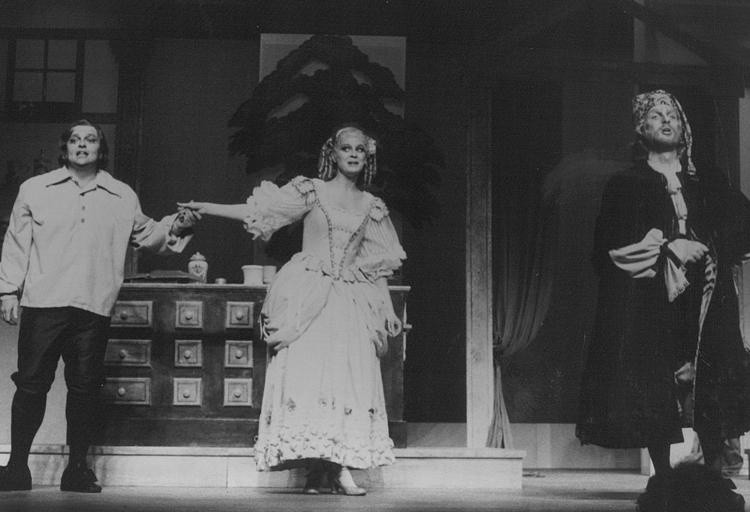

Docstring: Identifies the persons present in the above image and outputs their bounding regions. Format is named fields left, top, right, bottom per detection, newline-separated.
left=0, top=117, right=204, bottom=494
left=634, top=459, right=746, bottom=511
left=571, top=86, right=750, bottom=476
left=175, top=124, right=410, bottom=498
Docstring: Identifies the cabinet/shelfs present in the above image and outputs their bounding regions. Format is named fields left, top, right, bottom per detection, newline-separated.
left=97, top=282, right=408, bottom=445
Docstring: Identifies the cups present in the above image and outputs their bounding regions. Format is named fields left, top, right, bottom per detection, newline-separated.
left=240, top=264, right=277, bottom=286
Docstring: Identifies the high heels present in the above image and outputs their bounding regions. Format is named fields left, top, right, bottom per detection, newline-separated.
left=303, top=470, right=324, bottom=495
left=328, top=467, right=367, bottom=495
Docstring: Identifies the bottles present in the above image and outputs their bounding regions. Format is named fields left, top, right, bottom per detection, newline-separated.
left=187, top=250, right=209, bottom=285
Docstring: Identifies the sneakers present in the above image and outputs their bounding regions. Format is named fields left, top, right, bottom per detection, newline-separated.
left=60, top=466, right=102, bottom=493
left=2, top=464, right=33, bottom=491
